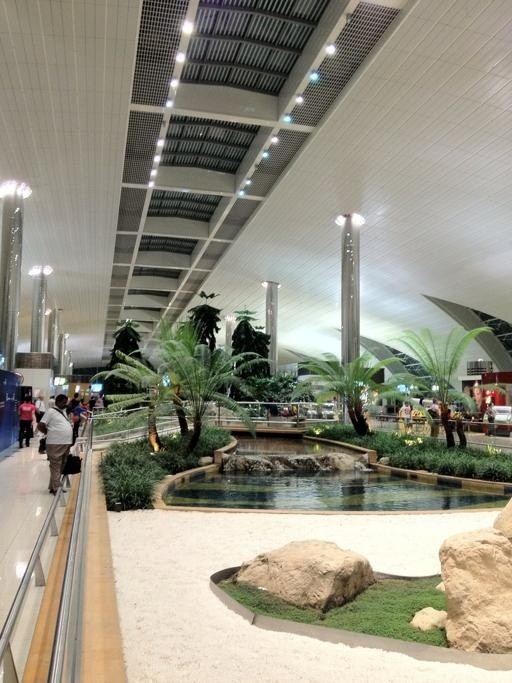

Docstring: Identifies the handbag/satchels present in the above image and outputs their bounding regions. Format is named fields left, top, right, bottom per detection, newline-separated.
left=61, top=454, right=83, bottom=474
left=38, top=436, right=47, bottom=455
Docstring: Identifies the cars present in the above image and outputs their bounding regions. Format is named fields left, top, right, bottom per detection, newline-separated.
left=307, top=404, right=334, bottom=418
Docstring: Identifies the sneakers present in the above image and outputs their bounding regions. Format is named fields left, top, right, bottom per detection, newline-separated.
left=50, top=488, right=68, bottom=495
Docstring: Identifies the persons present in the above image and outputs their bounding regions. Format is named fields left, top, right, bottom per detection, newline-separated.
left=17, top=392, right=104, bottom=447
left=37, top=394, right=73, bottom=493
left=485, top=401, right=497, bottom=435
left=399, top=400, right=414, bottom=434
left=430, top=396, right=441, bottom=436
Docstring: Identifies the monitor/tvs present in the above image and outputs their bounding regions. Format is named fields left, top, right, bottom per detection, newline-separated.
left=91, top=383, right=103, bottom=393
